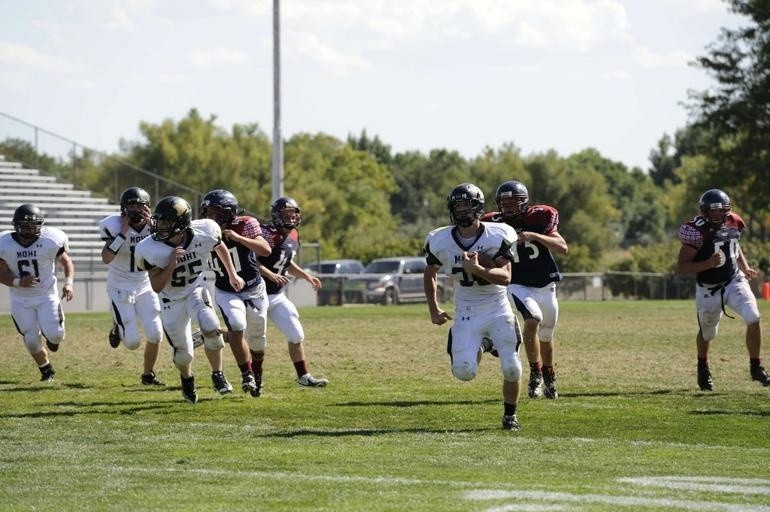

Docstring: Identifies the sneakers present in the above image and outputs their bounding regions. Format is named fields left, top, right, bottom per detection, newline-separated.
left=541, top=367, right=558, bottom=399
left=502, top=416, right=520, bottom=431
left=528, top=372, right=544, bottom=398
left=481, top=332, right=499, bottom=357
left=110, top=319, right=330, bottom=404
left=751, top=367, right=770, bottom=387
left=47, top=339, right=60, bottom=352
left=40, top=370, right=56, bottom=381
left=698, top=373, right=714, bottom=390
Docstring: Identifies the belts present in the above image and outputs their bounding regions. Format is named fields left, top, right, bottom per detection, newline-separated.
left=696, top=268, right=740, bottom=319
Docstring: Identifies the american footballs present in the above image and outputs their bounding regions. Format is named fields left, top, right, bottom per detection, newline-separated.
left=467, top=250, right=498, bottom=269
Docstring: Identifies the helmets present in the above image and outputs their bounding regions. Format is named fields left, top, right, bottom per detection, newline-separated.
left=446, top=183, right=485, bottom=228
left=12, top=204, right=43, bottom=238
left=699, top=189, right=732, bottom=228
left=495, top=181, right=529, bottom=216
left=120, top=187, right=302, bottom=242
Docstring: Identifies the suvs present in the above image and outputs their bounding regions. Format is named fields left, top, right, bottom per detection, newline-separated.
left=305, top=257, right=363, bottom=304
left=364, top=258, right=452, bottom=305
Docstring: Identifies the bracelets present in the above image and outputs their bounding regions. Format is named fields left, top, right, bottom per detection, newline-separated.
left=62, top=276, right=73, bottom=285
left=12, top=278, right=21, bottom=287
left=108, top=234, right=126, bottom=256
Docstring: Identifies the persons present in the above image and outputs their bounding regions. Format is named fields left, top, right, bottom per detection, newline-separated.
left=200, top=190, right=273, bottom=395
left=676, top=189, right=770, bottom=390
left=421, top=183, right=523, bottom=433
left=134, top=197, right=246, bottom=405
left=0, top=205, right=74, bottom=386
left=190, top=199, right=330, bottom=389
left=99, top=187, right=168, bottom=386
left=479, top=181, right=568, bottom=399
